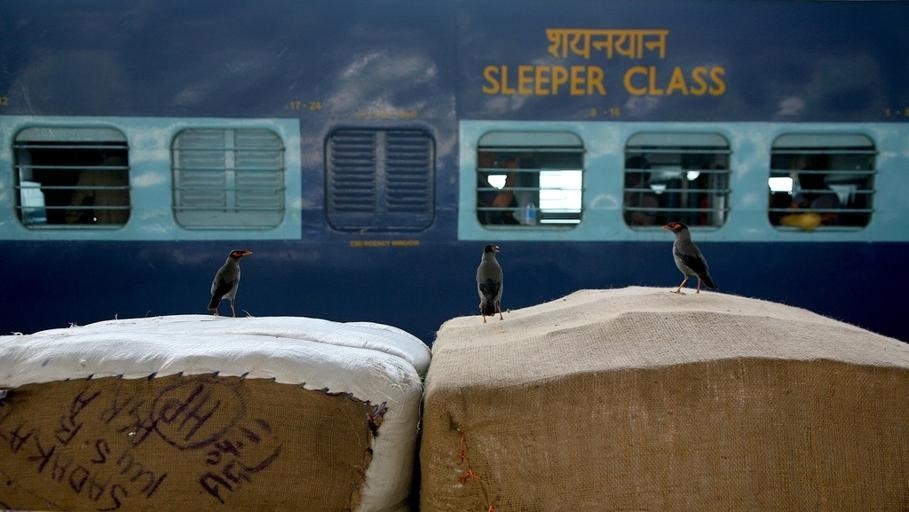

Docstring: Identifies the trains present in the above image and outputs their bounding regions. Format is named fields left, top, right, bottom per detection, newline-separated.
left=0, top=2, right=908, bottom=344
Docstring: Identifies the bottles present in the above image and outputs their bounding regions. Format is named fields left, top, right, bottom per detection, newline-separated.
left=520, top=200, right=536, bottom=225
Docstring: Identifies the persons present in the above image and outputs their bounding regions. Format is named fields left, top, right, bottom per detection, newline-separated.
left=476, top=145, right=520, bottom=226
left=770, top=164, right=841, bottom=227
left=623, top=156, right=727, bottom=226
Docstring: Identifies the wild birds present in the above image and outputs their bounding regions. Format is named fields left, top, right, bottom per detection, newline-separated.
left=476, top=245, right=505, bottom=324
left=208, top=249, right=253, bottom=317
left=661, top=221, right=716, bottom=296
